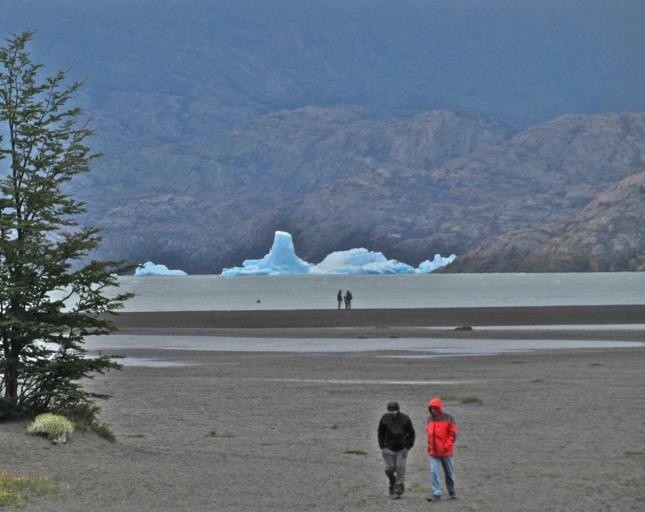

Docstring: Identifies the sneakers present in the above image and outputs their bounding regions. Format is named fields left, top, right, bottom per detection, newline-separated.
left=427, top=495, right=440, bottom=502
left=389, top=483, right=404, bottom=494
left=449, top=489, right=456, bottom=498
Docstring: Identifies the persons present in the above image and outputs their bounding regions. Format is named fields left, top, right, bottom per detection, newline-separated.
left=337, top=290, right=342, bottom=311
left=424, top=397, right=459, bottom=501
left=378, top=401, right=416, bottom=499
left=344, top=291, right=353, bottom=312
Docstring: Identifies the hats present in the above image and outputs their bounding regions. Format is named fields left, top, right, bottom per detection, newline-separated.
left=387, top=403, right=399, bottom=411
